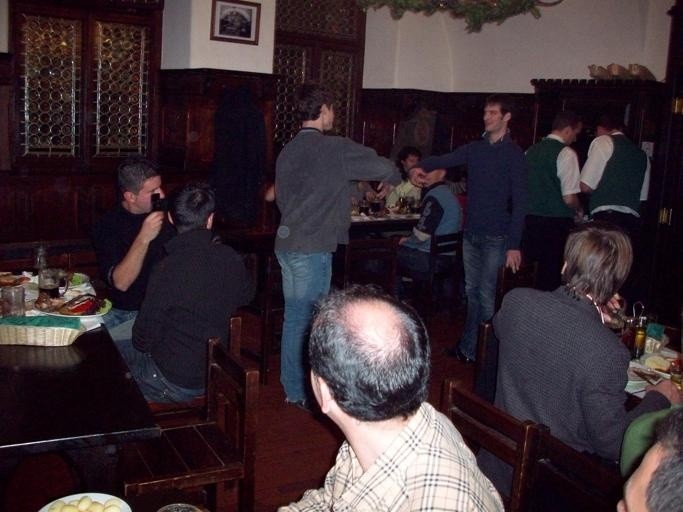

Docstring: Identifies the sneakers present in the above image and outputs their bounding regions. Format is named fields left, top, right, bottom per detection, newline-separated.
left=445, top=345, right=475, bottom=365
left=287, top=398, right=315, bottom=412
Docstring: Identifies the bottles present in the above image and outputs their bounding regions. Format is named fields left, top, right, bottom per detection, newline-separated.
left=622, top=316, right=648, bottom=359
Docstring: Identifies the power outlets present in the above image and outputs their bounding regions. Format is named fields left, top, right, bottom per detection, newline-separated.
left=641, top=140, right=655, bottom=158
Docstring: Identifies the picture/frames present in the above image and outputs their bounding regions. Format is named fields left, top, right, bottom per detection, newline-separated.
left=209, top=0, right=261, bottom=46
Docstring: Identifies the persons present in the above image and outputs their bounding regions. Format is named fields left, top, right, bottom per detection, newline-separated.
left=477, top=218, right=683, bottom=512
left=113, top=179, right=256, bottom=404
left=277, top=282, right=505, bottom=512
left=580, top=113, right=647, bottom=231
left=616, top=407, right=683, bottom=512
left=525, top=110, right=585, bottom=290
left=63, top=157, right=179, bottom=340
left=265, top=145, right=468, bottom=302
left=274, top=84, right=403, bottom=417
left=407, top=92, right=529, bottom=363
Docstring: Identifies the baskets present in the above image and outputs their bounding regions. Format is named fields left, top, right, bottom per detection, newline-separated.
left=1, top=316, right=87, bottom=348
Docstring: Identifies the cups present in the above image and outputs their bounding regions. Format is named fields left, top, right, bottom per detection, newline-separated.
left=0, top=285, right=26, bottom=317
left=670, top=359, right=683, bottom=391
left=601, top=296, right=628, bottom=329
left=30, top=240, right=71, bottom=300
left=157, top=502, right=202, bottom=512
left=357, top=199, right=369, bottom=216
left=371, top=200, right=381, bottom=213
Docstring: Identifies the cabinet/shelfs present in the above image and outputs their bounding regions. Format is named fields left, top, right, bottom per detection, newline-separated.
left=528, top=75, right=665, bottom=210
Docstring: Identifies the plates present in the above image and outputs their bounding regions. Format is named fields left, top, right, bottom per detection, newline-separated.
left=386, top=214, right=421, bottom=218
left=37, top=492, right=132, bottom=512
left=629, top=360, right=671, bottom=380
left=30, top=270, right=109, bottom=317
